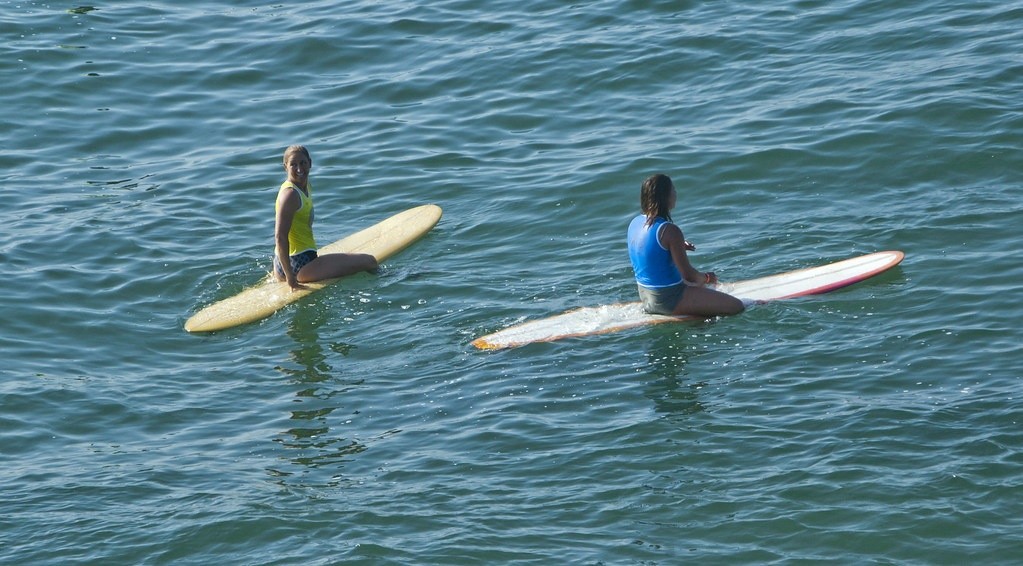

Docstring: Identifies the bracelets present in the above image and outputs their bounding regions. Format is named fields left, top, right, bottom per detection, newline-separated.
left=703, top=271, right=710, bottom=283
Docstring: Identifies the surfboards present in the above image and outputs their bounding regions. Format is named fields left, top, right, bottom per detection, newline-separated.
left=469, top=247, right=906, bottom=355
left=181, top=202, right=444, bottom=336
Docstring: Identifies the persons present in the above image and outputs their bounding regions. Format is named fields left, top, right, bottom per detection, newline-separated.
left=273, top=145, right=383, bottom=292
left=627, top=174, right=746, bottom=316
